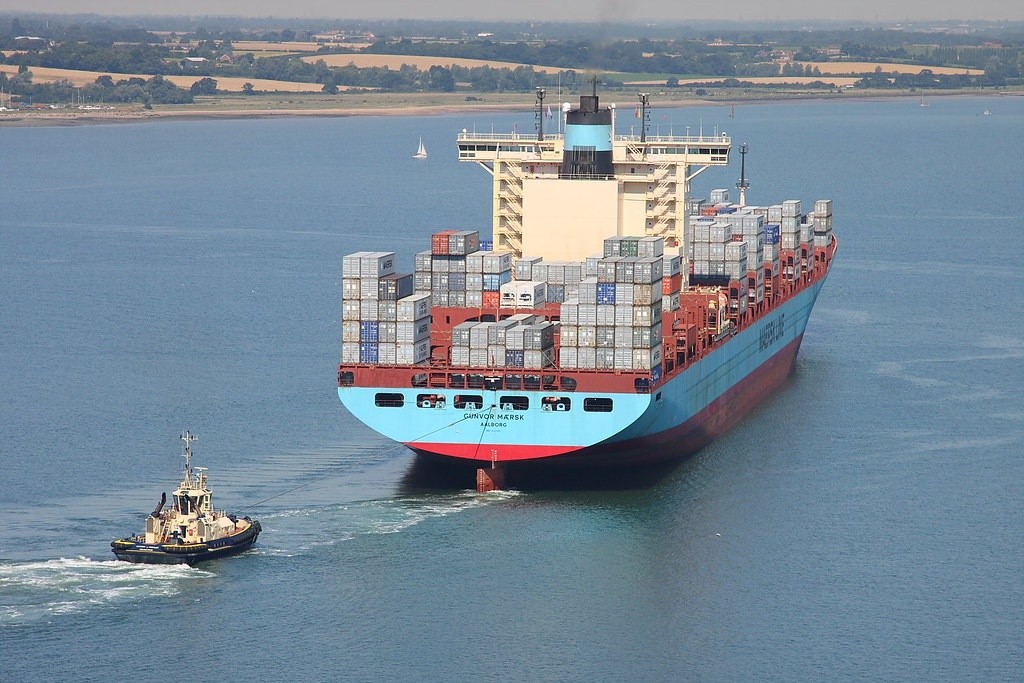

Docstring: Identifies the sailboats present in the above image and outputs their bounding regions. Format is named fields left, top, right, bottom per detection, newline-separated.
left=412, top=136, right=428, bottom=160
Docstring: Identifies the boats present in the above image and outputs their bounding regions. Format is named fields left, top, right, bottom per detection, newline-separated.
left=111, top=431, right=263, bottom=565
left=337, top=70, right=839, bottom=495
